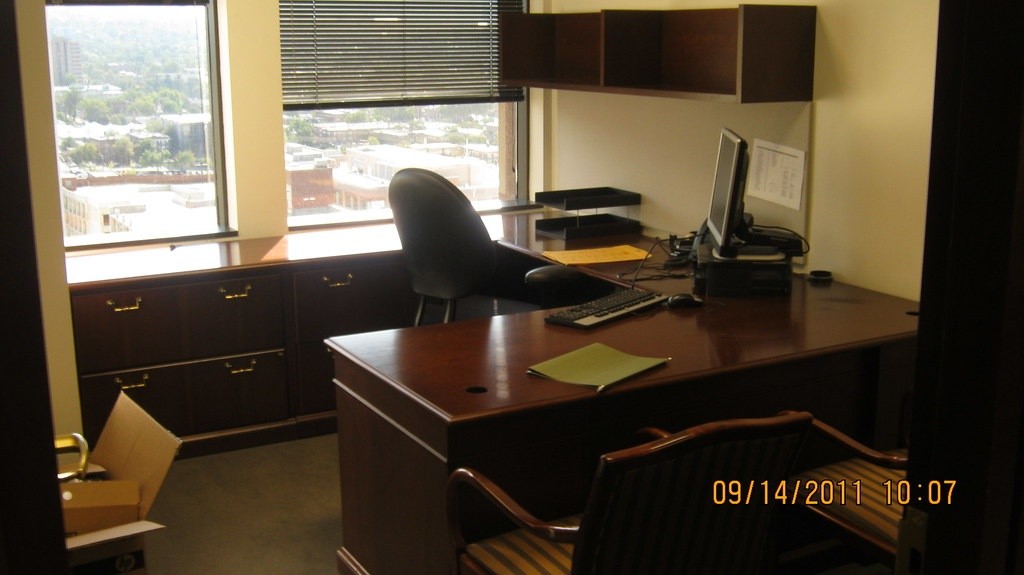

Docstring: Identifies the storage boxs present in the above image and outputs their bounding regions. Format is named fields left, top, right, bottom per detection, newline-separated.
left=54, top=390, right=182, bottom=575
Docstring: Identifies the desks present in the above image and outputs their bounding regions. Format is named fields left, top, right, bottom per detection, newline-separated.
left=325, top=225, right=918, bottom=575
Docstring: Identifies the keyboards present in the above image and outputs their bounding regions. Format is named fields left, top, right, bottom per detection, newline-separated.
left=544, top=288, right=668, bottom=330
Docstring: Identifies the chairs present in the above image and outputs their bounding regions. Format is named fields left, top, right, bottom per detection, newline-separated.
left=449, top=413, right=929, bottom=575
left=385, top=168, right=577, bottom=330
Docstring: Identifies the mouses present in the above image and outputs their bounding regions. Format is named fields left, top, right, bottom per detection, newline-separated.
left=668, top=293, right=704, bottom=309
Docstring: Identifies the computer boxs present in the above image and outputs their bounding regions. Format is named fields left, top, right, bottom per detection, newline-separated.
left=693, top=244, right=793, bottom=300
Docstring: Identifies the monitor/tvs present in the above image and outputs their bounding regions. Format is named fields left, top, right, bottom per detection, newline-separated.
left=706, top=128, right=804, bottom=260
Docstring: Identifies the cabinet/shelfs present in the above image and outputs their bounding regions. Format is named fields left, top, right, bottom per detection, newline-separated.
left=62, top=203, right=589, bottom=468
left=498, top=5, right=816, bottom=103
